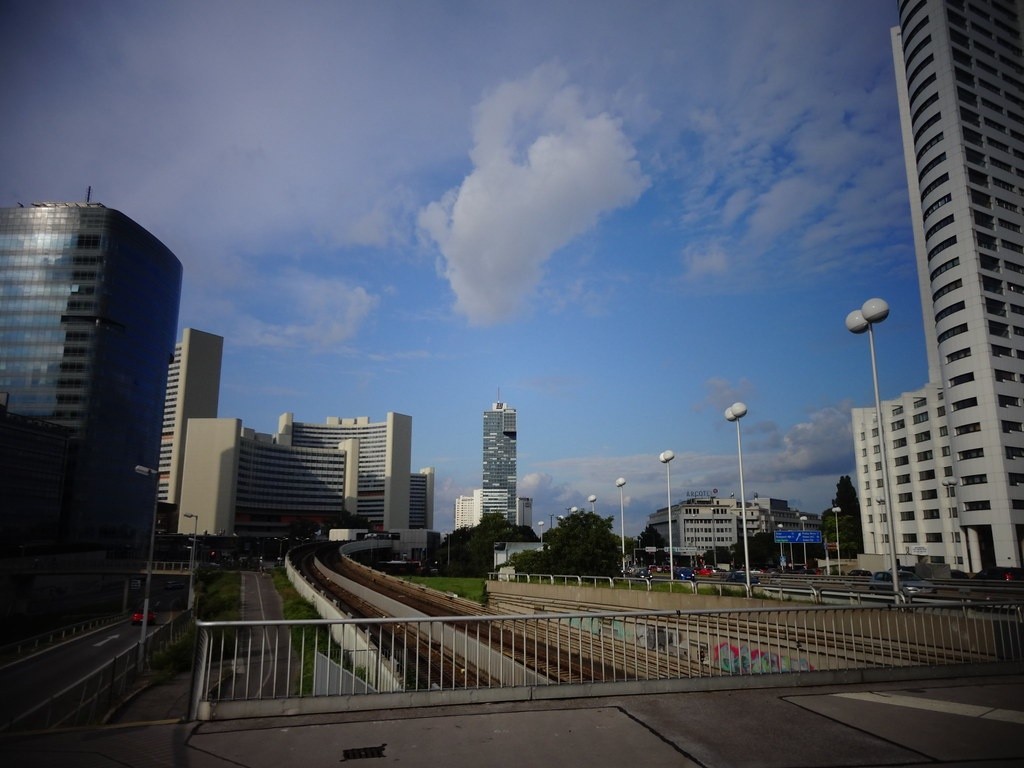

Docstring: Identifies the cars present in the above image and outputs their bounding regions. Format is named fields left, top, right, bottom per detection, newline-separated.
left=964, top=566, right=1024, bottom=609
left=130, top=606, right=156, bottom=625
left=622, top=564, right=970, bottom=607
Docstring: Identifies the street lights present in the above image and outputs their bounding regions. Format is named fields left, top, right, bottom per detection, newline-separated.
left=942, top=477, right=961, bottom=568
left=134, top=464, right=160, bottom=671
left=274, top=537, right=288, bottom=558
left=659, top=449, right=674, bottom=584
left=616, top=476, right=626, bottom=581
left=724, top=402, right=754, bottom=597
left=799, top=515, right=809, bottom=575
left=832, top=506, right=843, bottom=576
left=182, top=512, right=200, bottom=612
left=295, top=537, right=309, bottom=544
left=778, top=523, right=785, bottom=571
left=538, top=520, right=545, bottom=546
left=549, top=513, right=554, bottom=528
left=565, top=506, right=570, bottom=515
left=845, top=297, right=902, bottom=611
left=710, top=507, right=716, bottom=568
left=587, top=495, right=597, bottom=515
